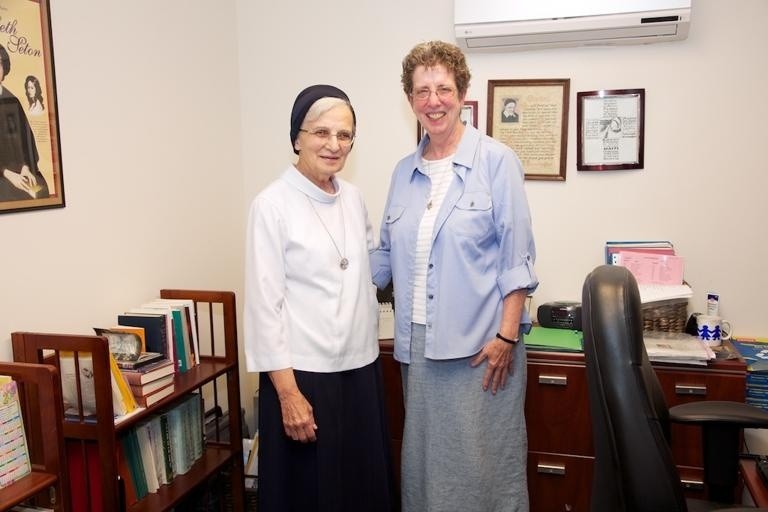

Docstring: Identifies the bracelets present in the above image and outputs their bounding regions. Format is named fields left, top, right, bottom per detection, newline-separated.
left=495, top=331, right=522, bottom=343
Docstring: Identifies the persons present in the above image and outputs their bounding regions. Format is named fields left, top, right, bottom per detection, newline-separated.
left=501, top=97, right=521, bottom=125
left=244, top=84, right=402, bottom=510
left=365, top=41, right=539, bottom=511
left=25, top=74, right=44, bottom=115
left=1, top=48, right=50, bottom=201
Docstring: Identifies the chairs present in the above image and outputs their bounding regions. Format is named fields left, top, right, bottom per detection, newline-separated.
left=582, top=265, right=768, bottom=512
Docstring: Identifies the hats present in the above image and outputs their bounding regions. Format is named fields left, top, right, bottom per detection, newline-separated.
left=290, top=85, right=356, bottom=155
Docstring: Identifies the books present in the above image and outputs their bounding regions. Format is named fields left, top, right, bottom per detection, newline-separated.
left=730, top=336, right=768, bottom=409
left=59, top=298, right=207, bottom=511
left=605, top=240, right=684, bottom=286
left=0, top=375, right=32, bottom=492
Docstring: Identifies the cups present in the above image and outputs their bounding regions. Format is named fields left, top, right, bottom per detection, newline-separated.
left=696, top=315, right=733, bottom=347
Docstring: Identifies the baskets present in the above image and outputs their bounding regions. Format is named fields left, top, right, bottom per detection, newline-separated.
left=222, top=487, right=258, bottom=512
left=642, top=281, right=690, bottom=333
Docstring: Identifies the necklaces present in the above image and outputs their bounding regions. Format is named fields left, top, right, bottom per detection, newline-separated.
left=306, top=197, right=352, bottom=271
left=428, top=144, right=453, bottom=208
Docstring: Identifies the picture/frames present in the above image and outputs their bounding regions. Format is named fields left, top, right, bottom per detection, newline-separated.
left=0, top=0, right=66, bottom=215
left=486, top=78, right=570, bottom=181
left=417, top=101, right=478, bottom=147
left=577, top=88, right=645, bottom=171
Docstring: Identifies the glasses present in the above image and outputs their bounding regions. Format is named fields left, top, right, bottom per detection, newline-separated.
left=298, top=128, right=355, bottom=145
left=410, top=85, right=456, bottom=99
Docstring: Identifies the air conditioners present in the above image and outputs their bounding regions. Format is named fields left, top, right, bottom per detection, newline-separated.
left=453, top=0, right=692, bottom=55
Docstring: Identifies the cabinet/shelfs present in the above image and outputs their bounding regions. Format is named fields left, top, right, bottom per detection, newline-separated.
left=11, top=288, right=246, bottom=512
left=378, top=337, right=748, bottom=512
left=0, top=361, right=72, bottom=512
